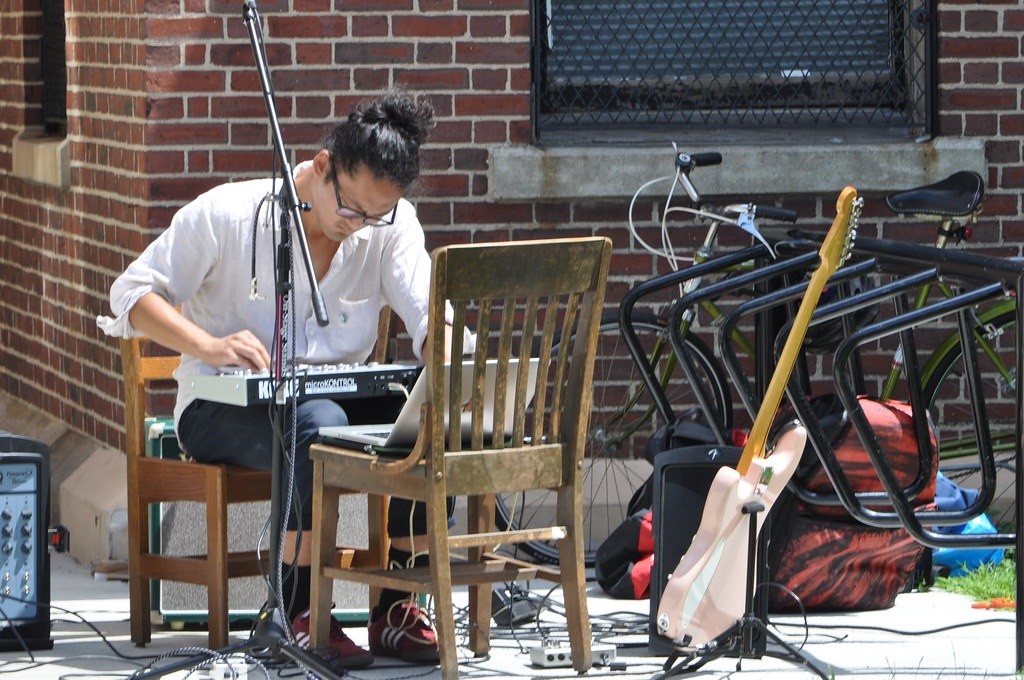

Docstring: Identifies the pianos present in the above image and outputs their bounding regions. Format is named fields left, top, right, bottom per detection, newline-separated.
left=188, top=359, right=428, bottom=408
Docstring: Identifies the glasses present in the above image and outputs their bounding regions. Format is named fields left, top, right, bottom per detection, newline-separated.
left=326, top=154, right=399, bottom=228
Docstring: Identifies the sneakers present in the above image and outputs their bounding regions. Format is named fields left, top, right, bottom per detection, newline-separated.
left=291, top=603, right=374, bottom=667
left=368, top=604, right=441, bottom=661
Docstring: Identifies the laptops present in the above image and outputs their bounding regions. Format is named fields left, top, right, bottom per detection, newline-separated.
left=318, top=358, right=554, bottom=447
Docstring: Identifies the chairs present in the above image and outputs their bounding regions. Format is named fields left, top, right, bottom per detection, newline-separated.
left=308, top=236, right=616, bottom=676
left=117, top=299, right=400, bottom=650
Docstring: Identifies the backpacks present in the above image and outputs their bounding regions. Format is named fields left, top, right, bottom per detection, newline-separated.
left=766, top=392, right=937, bottom=615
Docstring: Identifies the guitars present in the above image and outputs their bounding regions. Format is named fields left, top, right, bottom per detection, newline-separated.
left=654, top=183, right=865, bottom=654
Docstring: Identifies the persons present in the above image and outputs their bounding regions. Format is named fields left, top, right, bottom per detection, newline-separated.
left=96, top=87, right=480, bottom=669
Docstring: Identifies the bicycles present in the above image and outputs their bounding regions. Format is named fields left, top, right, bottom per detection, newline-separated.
left=482, top=148, right=1024, bottom=572
